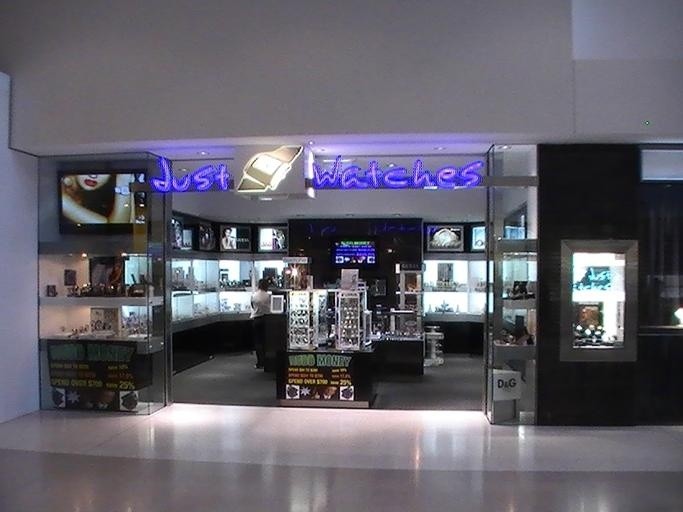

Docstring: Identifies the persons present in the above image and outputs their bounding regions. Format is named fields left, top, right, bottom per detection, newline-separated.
left=250, top=279, right=271, bottom=368
left=60, top=173, right=135, bottom=223
left=222, top=229, right=237, bottom=249
left=312, top=386, right=337, bottom=400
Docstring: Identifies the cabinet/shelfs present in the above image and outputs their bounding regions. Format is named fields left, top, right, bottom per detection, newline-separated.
left=38, top=176, right=537, bottom=360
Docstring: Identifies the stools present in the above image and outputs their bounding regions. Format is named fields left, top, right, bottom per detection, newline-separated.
left=424, top=325, right=444, bottom=368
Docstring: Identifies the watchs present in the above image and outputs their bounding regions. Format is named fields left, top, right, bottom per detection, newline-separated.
left=113, top=186, right=131, bottom=195
left=575, top=324, right=616, bottom=345
